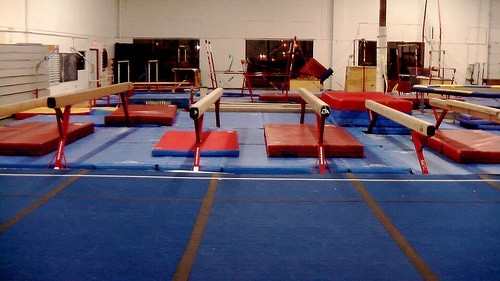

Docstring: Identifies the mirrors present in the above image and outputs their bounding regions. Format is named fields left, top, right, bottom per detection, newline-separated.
left=59, top=52, right=78, bottom=85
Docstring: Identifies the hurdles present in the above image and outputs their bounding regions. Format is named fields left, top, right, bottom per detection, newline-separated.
left=296, top=86, right=335, bottom=173
left=362, top=96, right=437, bottom=177
left=427, top=95, right=499, bottom=128
left=43, top=81, right=134, bottom=169
left=188, top=86, right=226, bottom=169
